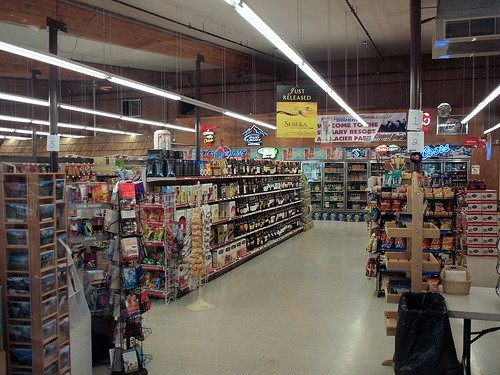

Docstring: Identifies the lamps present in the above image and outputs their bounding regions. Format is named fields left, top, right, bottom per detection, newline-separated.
left=460, top=18, right=500, bottom=134
left=223, top=0, right=369, bottom=128
left=0, top=0, right=276, bottom=141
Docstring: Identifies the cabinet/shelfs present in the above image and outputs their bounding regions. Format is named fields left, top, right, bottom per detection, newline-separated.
left=374, top=186, right=459, bottom=297
left=381, top=172, right=439, bottom=366
left=0, top=170, right=153, bottom=375
left=154, top=182, right=247, bottom=285
left=141, top=173, right=304, bottom=298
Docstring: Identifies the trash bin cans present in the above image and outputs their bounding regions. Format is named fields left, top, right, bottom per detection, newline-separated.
left=392, top=292, right=462, bottom=375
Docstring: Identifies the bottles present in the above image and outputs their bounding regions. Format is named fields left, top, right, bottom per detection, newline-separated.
left=235, top=190, right=300, bottom=216
left=238, top=176, right=302, bottom=194
left=183, top=159, right=302, bottom=175
left=420, top=164, right=488, bottom=190
left=233, top=203, right=301, bottom=251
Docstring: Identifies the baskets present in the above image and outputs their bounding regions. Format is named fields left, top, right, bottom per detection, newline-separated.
left=440, top=265, right=473, bottom=295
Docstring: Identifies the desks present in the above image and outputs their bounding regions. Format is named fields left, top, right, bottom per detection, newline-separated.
left=438, top=285, right=500, bottom=375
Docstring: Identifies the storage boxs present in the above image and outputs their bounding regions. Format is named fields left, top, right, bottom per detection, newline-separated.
left=462, top=189, right=498, bottom=256
